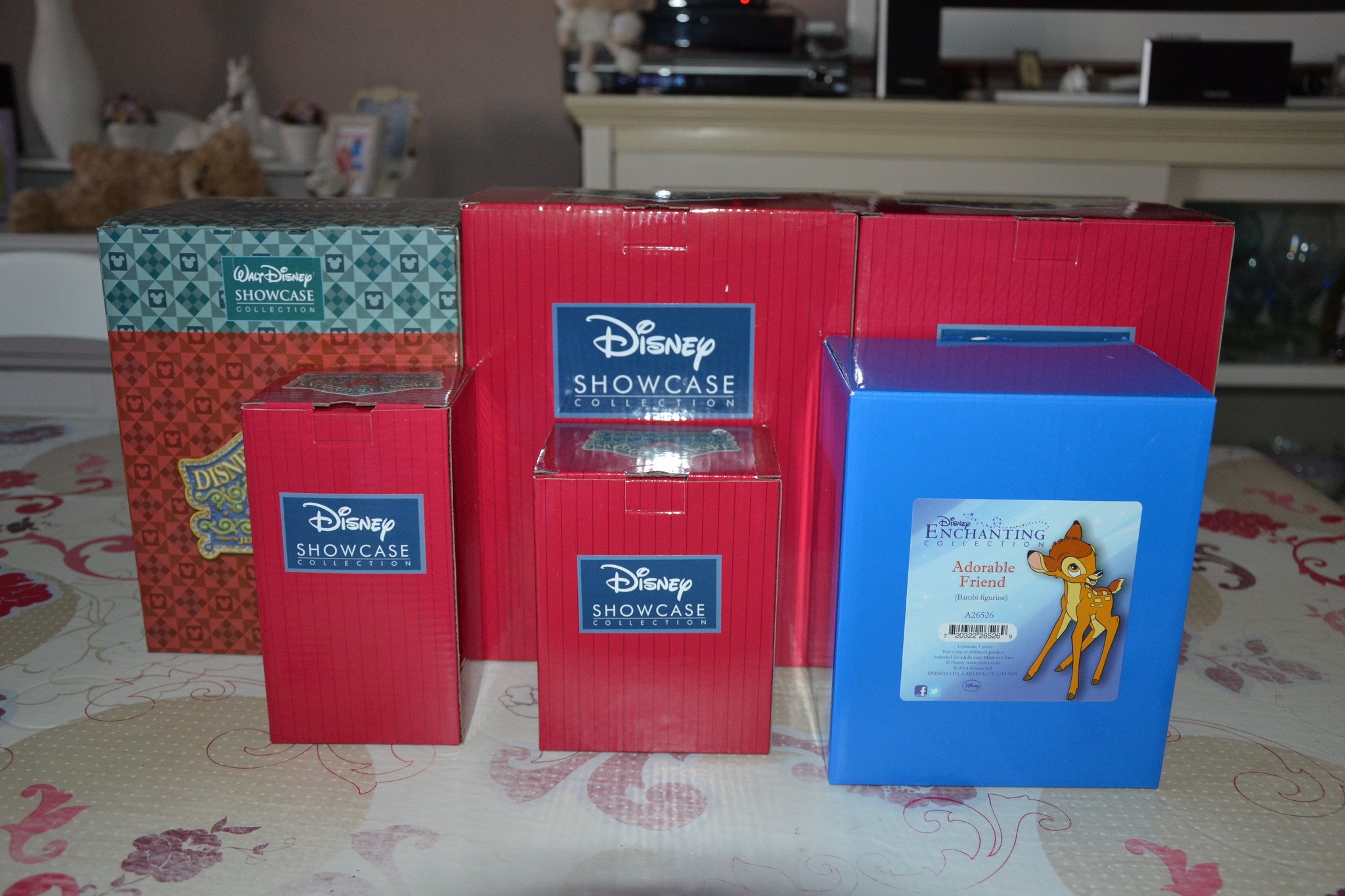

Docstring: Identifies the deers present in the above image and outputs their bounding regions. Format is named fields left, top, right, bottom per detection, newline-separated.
left=168, top=53, right=278, bottom=160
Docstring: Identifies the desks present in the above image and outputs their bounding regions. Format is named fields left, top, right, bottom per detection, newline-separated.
left=0, top=414, right=1345, bottom=894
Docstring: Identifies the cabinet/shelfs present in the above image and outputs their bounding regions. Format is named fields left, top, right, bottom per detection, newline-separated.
left=557, top=95, right=1343, bottom=388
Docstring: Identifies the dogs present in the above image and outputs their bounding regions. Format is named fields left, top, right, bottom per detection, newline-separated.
left=7, top=124, right=279, bottom=232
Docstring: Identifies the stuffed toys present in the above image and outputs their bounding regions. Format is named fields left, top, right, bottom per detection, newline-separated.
left=9, top=122, right=272, bottom=235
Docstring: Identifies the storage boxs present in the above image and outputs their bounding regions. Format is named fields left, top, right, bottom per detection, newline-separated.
left=241, top=368, right=486, bottom=747
left=458, top=180, right=858, bottom=672
left=853, top=180, right=1237, bottom=396
left=97, top=191, right=464, bottom=659
left=807, top=337, right=1218, bottom=792
left=531, top=421, right=783, bottom=756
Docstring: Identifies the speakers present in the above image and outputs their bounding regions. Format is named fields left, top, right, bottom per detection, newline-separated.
left=874, top=1, right=943, bottom=98
left=1138, top=36, right=1295, bottom=108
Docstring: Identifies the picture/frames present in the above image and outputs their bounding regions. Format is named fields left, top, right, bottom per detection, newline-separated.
left=306, top=115, right=382, bottom=200
left=351, top=86, right=420, bottom=190
